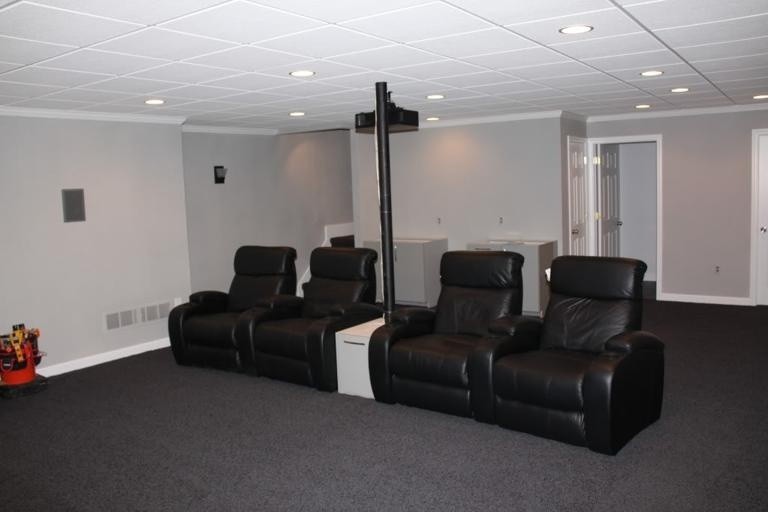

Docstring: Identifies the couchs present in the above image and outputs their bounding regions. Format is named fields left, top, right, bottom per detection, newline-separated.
left=495, top=255, right=665, bottom=455
left=167, top=245, right=297, bottom=374
left=252, top=244, right=383, bottom=397
left=369, top=250, right=524, bottom=425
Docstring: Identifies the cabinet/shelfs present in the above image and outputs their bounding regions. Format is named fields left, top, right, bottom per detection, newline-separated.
left=363, top=237, right=558, bottom=320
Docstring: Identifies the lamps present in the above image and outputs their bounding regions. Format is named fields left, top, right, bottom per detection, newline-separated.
left=354, top=92, right=420, bottom=134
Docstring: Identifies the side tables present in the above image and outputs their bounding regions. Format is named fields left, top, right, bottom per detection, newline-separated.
left=335, top=318, right=384, bottom=402
